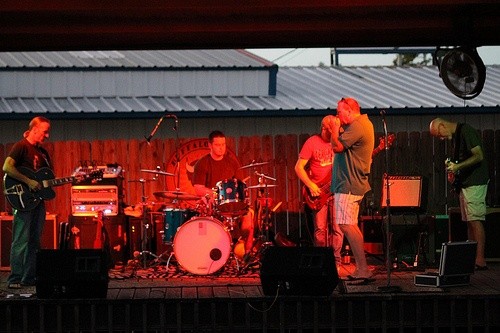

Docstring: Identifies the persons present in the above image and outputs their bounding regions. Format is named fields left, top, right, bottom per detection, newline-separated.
left=2, top=116, right=85, bottom=289
left=295, top=97, right=376, bottom=282
left=194, top=130, right=258, bottom=265
left=430, top=118, right=490, bottom=271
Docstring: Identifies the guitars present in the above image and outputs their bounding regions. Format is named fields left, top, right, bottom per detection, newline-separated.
left=3, top=166, right=103, bottom=211
left=302, top=133, right=396, bottom=211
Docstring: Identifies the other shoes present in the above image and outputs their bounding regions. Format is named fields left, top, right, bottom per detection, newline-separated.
left=244, top=253, right=257, bottom=262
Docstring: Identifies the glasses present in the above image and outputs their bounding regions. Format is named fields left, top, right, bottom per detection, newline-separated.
left=437, top=123, right=443, bottom=137
left=341, top=97, right=354, bottom=112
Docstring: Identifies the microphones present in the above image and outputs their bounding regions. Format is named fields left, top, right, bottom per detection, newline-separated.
left=380, top=110, right=386, bottom=122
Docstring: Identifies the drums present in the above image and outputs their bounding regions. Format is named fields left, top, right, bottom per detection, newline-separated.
left=171, top=215, right=234, bottom=277
left=162, top=209, right=200, bottom=245
left=215, top=178, right=248, bottom=212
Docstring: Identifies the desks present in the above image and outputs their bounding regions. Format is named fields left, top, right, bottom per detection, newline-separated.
left=0, top=212, right=58, bottom=270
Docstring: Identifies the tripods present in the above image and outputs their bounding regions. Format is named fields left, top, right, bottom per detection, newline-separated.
left=138, top=182, right=173, bottom=268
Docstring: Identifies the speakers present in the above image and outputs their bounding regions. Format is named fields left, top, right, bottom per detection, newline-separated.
left=447, top=207, right=500, bottom=261
left=36, top=248, right=111, bottom=296
left=380, top=175, right=422, bottom=207
left=261, top=245, right=340, bottom=295
left=0, top=216, right=56, bottom=271
left=70, top=216, right=127, bottom=250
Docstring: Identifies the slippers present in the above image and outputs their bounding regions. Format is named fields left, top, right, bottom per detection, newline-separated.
left=474, top=263, right=488, bottom=272
left=343, top=274, right=376, bottom=286
left=8, top=282, right=22, bottom=289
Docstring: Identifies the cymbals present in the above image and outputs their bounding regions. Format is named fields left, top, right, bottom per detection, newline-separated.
left=248, top=184, right=278, bottom=189
left=153, top=190, right=201, bottom=200
left=141, top=169, right=173, bottom=176
left=240, top=160, right=271, bottom=169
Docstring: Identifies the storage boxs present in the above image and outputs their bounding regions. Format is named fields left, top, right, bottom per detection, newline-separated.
left=413, top=240, right=478, bottom=288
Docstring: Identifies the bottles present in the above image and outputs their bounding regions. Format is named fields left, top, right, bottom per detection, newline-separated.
left=342, top=245, right=351, bottom=265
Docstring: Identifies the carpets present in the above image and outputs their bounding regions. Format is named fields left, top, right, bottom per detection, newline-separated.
left=109, top=263, right=259, bottom=278
